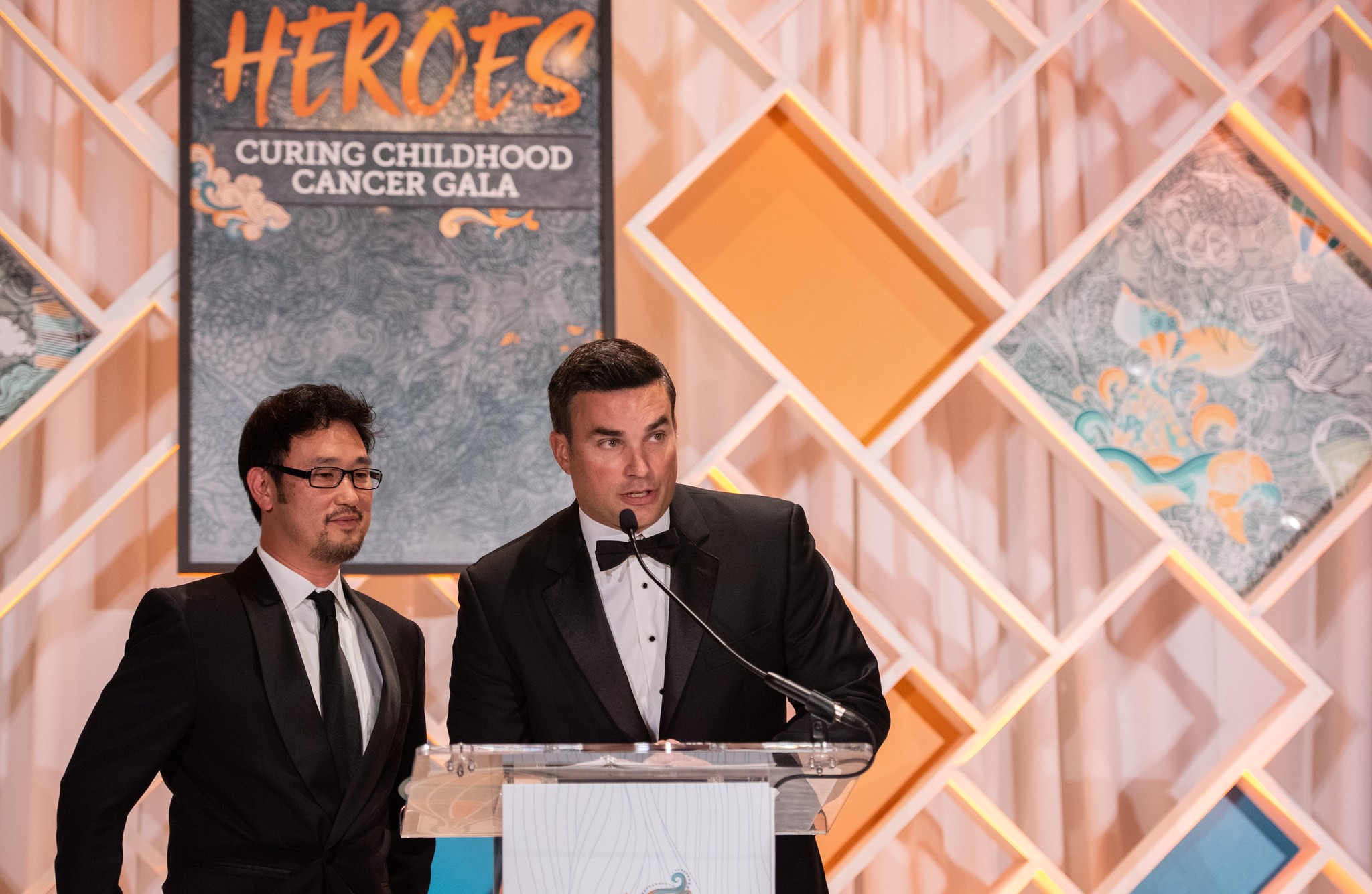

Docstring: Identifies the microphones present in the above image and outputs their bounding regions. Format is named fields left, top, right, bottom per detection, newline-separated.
left=620, top=509, right=869, bottom=730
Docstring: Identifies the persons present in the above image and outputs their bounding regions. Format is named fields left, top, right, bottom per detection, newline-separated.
left=447, top=338, right=890, bottom=894
left=54, top=384, right=437, bottom=894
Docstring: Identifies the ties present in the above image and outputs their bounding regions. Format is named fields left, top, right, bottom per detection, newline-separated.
left=306, top=590, right=364, bottom=802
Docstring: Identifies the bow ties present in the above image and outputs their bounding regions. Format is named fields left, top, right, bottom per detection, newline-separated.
left=596, top=528, right=682, bottom=572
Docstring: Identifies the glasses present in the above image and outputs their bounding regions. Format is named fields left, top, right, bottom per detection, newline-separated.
left=260, top=462, right=383, bottom=490
